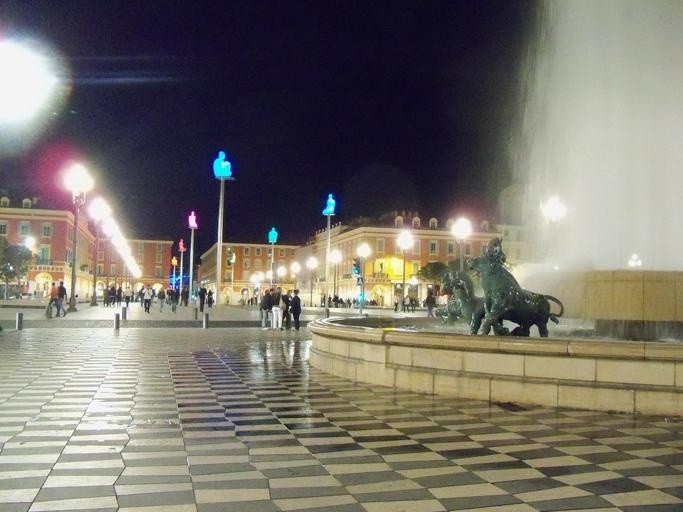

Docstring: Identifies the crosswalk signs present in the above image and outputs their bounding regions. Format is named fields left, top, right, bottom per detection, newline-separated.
left=357, top=278, right=362, bottom=286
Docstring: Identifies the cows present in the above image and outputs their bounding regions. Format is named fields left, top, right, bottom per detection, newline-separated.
left=465, top=238, right=565, bottom=336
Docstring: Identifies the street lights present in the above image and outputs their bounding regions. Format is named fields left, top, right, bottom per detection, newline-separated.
left=178, top=238, right=187, bottom=307
left=322, top=193, right=336, bottom=308
left=247, top=244, right=342, bottom=307
left=449, top=219, right=471, bottom=274
left=213, top=151, right=236, bottom=307
left=188, top=211, right=198, bottom=307
left=267, top=227, right=278, bottom=289
left=357, top=242, right=369, bottom=313
left=171, top=256, right=178, bottom=290
left=59, top=162, right=141, bottom=307
left=394, top=226, right=418, bottom=311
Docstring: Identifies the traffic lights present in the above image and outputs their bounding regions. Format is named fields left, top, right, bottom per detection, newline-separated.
left=352, top=258, right=360, bottom=275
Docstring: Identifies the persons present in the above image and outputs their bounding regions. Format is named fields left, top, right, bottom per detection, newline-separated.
left=45, top=282, right=58, bottom=316
left=103, top=285, right=214, bottom=313
left=58, top=281, right=67, bottom=317
left=424, top=292, right=435, bottom=318
left=323, top=295, right=419, bottom=313
left=248, top=287, right=301, bottom=331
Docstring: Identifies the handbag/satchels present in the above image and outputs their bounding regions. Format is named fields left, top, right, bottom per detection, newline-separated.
left=278, top=299, right=286, bottom=311
